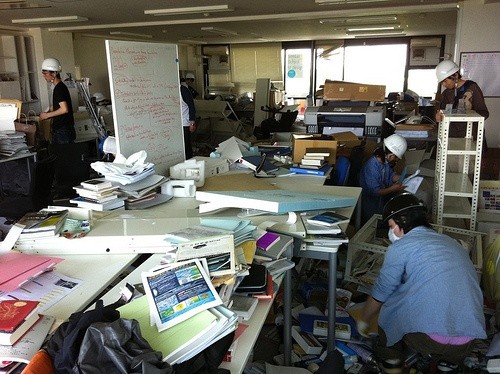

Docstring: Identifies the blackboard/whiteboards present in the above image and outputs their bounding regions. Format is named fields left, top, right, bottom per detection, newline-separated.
left=106, top=40, right=186, bottom=176
left=459, top=51, right=500, bottom=98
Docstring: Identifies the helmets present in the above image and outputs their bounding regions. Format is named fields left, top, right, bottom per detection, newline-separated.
left=383, top=193, right=424, bottom=222
left=42, top=57, right=62, bottom=71
left=435, top=60, right=460, bottom=83
left=384, top=134, right=407, bottom=159
left=186, top=73, right=195, bottom=79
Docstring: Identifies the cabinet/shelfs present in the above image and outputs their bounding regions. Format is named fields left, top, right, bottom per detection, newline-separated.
left=195, top=99, right=246, bottom=138
left=254, top=78, right=285, bottom=128
left=345, top=110, right=485, bottom=291
left=202, top=46, right=234, bottom=95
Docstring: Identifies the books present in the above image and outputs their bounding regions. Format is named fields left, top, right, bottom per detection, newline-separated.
left=289, top=148, right=333, bottom=178
left=116, top=211, right=350, bottom=365
left=0, top=300, right=64, bottom=365
left=11, top=164, right=168, bottom=251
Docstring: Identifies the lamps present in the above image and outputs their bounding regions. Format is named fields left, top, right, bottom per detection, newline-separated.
left=144, top=4, right=237, bottom=16
left=319, top=15, right=405, bottom=35
left=11, top=14, right=88, bottom=25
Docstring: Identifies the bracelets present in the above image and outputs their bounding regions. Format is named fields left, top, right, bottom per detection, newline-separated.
left=191, top=124, right=194, bottom=126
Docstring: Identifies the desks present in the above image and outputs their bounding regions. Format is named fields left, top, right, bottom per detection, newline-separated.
left=0, top=136, right=362, bottom=374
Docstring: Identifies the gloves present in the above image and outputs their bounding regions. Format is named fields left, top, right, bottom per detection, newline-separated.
left=356, top=321, right=370, bottom=339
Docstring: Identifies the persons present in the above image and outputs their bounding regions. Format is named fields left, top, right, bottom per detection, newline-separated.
left=358, top=134, right=405, bottom=228
left=357, top=192, right=487, bottom=374
left=26, top=58, right=89, bottom=212
left=180, top=73, right=200, bottom=160
left=434, top=59, right=489, bottom=164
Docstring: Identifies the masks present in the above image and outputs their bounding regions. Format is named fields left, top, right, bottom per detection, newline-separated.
left=388, top=225, right=403, bottom=243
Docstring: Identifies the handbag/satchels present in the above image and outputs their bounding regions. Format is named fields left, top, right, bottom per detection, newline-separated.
left=13, top=111, right=39, bottom=148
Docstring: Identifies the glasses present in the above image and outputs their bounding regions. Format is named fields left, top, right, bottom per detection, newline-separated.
left=42, top=73, right=51, bottom=77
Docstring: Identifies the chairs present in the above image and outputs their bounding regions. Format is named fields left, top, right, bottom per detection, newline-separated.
left=260, top=105, right=286, bottom=133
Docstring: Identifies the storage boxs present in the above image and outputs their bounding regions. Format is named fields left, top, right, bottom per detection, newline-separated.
left=333, top=132, right=362, bottom=153
left=292, top=134, right=337, bottom=165
left=324, top=79, right=386, bottom=101
left=313, top=319, right=351, bottom=340
left=478, top=180, right=500, bottom=244
left=291, top=325, right=323, bottom=355
left=28, top=108, right=101, bottom=143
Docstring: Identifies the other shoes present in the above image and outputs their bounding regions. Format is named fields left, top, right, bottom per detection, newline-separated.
left=379, top=359, right=402, bottom=374
left=437, top=356, right=460, bottom=374
left=49, top=192, right=71, bottom=203
